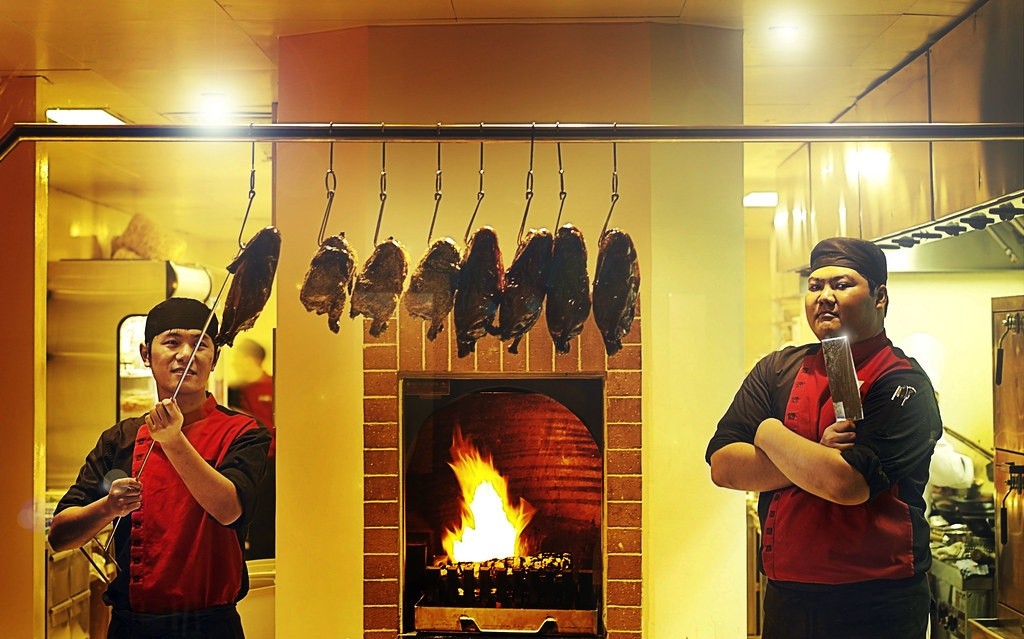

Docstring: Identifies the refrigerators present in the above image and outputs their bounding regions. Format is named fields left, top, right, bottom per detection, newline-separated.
left=46, top=258, right=212, bottom=493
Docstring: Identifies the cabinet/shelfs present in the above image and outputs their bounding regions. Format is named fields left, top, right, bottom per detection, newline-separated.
left=768, top=0, right=1023, bottom=272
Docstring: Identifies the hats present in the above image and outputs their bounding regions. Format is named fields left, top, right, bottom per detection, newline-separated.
left=145, top=297, right=218, bottom=359
left=810, top=237, right=888, bottom=288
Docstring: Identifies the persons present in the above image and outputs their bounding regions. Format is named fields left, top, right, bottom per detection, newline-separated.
left=48, top=297, right=272, bottom=639
left=704, top=236, right=943, bottom=639
left=227, top=339, right=275, bottom=560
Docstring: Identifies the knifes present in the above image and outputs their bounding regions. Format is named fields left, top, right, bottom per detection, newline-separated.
left=822, top=336, right=864, bottom=425
